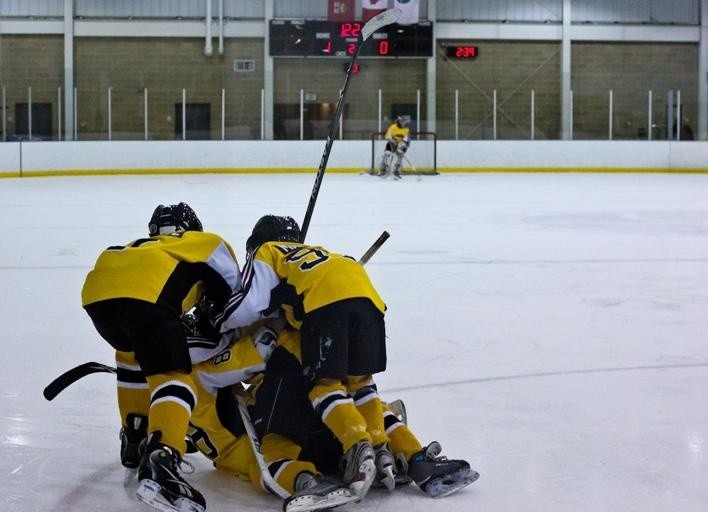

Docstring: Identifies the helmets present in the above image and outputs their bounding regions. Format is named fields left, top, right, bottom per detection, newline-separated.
left=246, top=214, right=305, bottom=253
left=148, top=202, right=203, bottom=237
left=396, top=115, right=408, bottom=124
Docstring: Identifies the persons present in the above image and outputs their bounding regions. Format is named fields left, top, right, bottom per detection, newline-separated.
left=212, top=214, right=397, bottom=498
left=81, top=201, right=240, bottom=512
left=378, top=115, right=412, bottom=179
left=186, top=314, right=480, bottom=512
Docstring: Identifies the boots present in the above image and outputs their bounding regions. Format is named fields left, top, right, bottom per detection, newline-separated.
left=377, top=168, right=403, bottom=180
left=282, top=439, right=471, bottom=512
left=119, top=412, right=207, bottom=512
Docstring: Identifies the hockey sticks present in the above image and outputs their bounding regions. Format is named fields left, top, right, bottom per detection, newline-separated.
left=399, top=148, right=420, bottom=182
left=43, top=362, right=117, bottom=401
left=236, top=394, right=292, bottom=499
